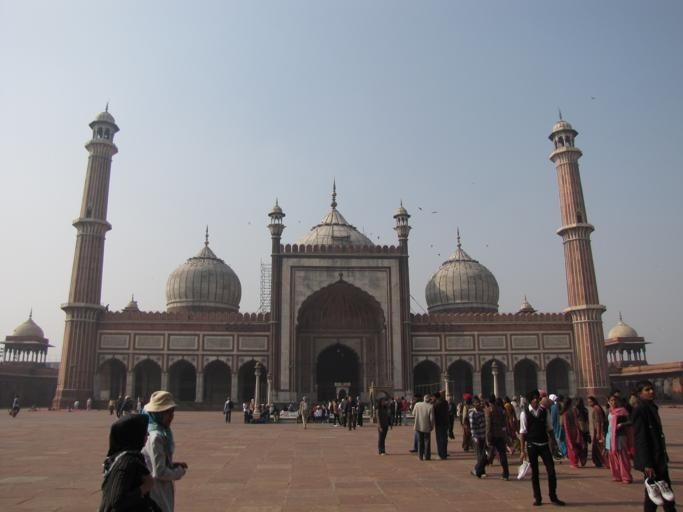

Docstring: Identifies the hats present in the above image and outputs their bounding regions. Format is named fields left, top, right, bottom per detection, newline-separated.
left=143, top=389, right=180, bottom=415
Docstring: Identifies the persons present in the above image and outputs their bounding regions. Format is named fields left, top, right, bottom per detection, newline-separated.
left=409, top=380, right=676, bottom=511
left=142, top=391, right=188, bottom=511
left=223, top=397, right=234, bottom=423
left=243, top=397, right=280, bottom=424
left=86, top=396, right=92, bottom=412
left=284, top=396, right=418, bottom=429
left=11, top=395, right=21, bottom=417
left=109, top=394, right=148, bottom=418
left=375, top=398, right=392, bottom=455
left=99, top=414, right=163, bottom=512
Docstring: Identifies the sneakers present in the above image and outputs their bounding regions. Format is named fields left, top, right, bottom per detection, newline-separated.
left=516, top=460, right=532, bottom=482
left=532, top=497, right=542, bottom=506
left=550, top=498, right=566, bottom=506
left=654, top=479, right=674, bottom=502
left=644, top=477, right=665, bottom=508
left=377, top=447, right=516, bottom=481
